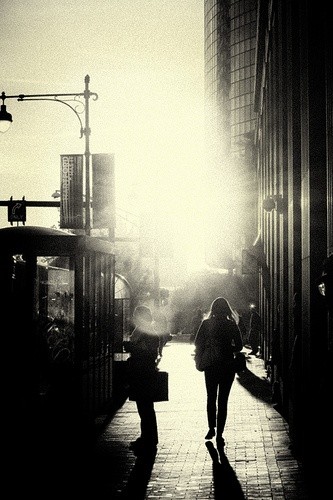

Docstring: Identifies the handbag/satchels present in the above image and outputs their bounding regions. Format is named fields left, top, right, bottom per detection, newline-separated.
left=196, top=336, right=218, bottom=370
left=126, top=371, right=169, bottom=401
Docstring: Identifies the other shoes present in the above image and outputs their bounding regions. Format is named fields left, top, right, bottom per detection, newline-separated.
left=131, top=438, right=145, bottom=447
left=218, top=437, right=225, bottom=443
left=206, top=430, right=216, bottom=439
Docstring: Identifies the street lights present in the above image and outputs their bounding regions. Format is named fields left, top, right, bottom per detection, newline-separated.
left=0, top=91, right=91, bottom=234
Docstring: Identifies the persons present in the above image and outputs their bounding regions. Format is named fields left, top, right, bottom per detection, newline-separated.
left=248, top=307, right=259, bottom=355
left=195, top=296, right=243, bottom=442
left=127, top=306, right=160, bottom=444
left=153, top=305, right=167, bottom=357
